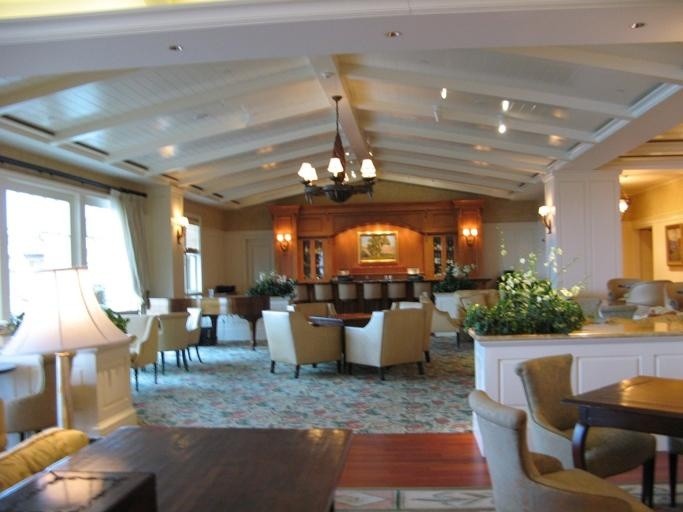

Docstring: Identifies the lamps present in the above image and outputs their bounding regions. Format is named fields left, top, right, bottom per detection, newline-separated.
left=176, top=215, right=188, bottom=242
left=462, top=228, right=478, bottom=246
left=619, top=198, right=630, bottom=220
left=538, top=205, right=555, bottom=232
left=296, top=95, right=378, bottom=204
left=276, top=231, right=291, bottom=252
left=0, top=270, right=138, bottom=430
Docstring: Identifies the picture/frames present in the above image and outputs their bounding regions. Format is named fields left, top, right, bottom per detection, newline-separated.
left=663, top=222, right=682, bottom=265
left=357, top=230, right=398, bottom=266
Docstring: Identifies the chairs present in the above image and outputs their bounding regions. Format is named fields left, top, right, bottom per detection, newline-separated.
left=626, top=280, right=672, bottom=306
left=663, top=282, right=682, bottom=312
left=465, top=388, right=655, bottom=511
left=420, top=275, right=504, bottom=348
left=607, top=279, right=643, bottom=304
left=0, top=350, right=80, bottom=443
left=512, top=352, right=657, bottom=510
left=262, top=281, right=432, bottom=383
left=129, top=306, right=206, bottom=382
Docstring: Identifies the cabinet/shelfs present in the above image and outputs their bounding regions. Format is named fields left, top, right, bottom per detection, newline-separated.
left=298, top=239, right=327, bottom=281
left=432, top=236, right=455, bottom=274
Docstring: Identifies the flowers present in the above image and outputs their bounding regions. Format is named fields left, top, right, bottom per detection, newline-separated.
left=462, top=270, right=587, bottom=336
left=248, top=271, right=294, bottom=298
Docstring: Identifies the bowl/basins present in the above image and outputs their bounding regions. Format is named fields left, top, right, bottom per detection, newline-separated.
left=336, top=270, right=349, bottom=276
left=407, top=267, right=418, bottom=274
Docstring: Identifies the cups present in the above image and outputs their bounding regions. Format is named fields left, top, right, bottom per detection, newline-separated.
left=206, top=288, right=213, bottom=297
